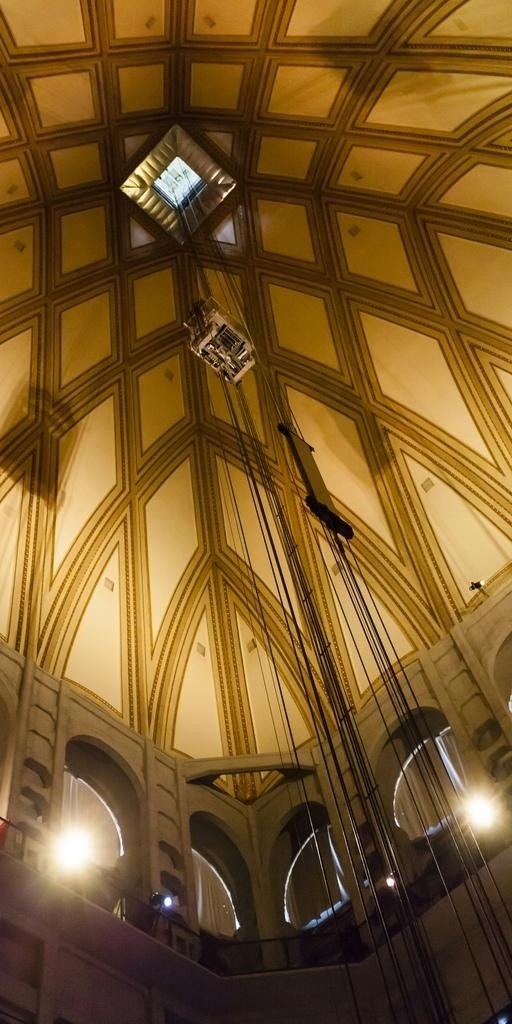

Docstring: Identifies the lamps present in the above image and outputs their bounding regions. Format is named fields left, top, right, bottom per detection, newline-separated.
left=149, top=891, right=173, bottom=937
